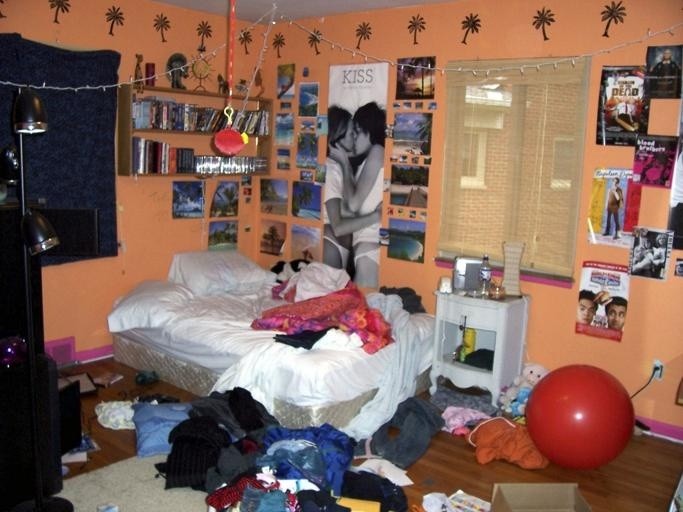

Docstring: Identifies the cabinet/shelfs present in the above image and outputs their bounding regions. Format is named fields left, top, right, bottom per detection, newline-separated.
left=117, top=84, right=274, bottom=178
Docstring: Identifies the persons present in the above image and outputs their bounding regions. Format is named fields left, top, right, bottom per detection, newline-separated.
left=324, top=106, right=386, bottom=274
left=607, top=296, right=627, bottom=328
left=650, top=49, right=680, bottom=98
left=576, top=290, right=597, bottom=326
left=604, top=180, right=624, bottom=240
left=349, top=103, right=383, bottom=287
left=632, top=234, right=668, bottom=278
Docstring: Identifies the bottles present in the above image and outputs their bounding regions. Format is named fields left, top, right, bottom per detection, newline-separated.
left=490, top=271, right=505, bottom=302
left=463, top=327, right=475, bottom=361
left=479, top=255, right=491, bottom=297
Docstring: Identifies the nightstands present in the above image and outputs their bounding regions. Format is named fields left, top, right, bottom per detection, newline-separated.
left=430, top=287, right=531, bottom=407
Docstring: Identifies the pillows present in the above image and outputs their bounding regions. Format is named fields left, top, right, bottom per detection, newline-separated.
left=179, top=250, right=268, bottom=296
left=106, top=280, right=195, bottom=334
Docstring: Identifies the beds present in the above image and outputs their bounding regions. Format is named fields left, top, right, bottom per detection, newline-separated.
left=112, top=284, right=436, bottom=427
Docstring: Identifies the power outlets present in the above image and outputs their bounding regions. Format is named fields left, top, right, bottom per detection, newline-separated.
left=653, top=359, right=664, bottom=381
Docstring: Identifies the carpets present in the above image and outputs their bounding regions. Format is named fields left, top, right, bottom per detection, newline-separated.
left=51, top=454, right=209, bottom=512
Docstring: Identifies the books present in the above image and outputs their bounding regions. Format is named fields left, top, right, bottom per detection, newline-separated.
left=131, top=139, right=193, bottom=175
left=134, top=98, right=269, bottom=135
